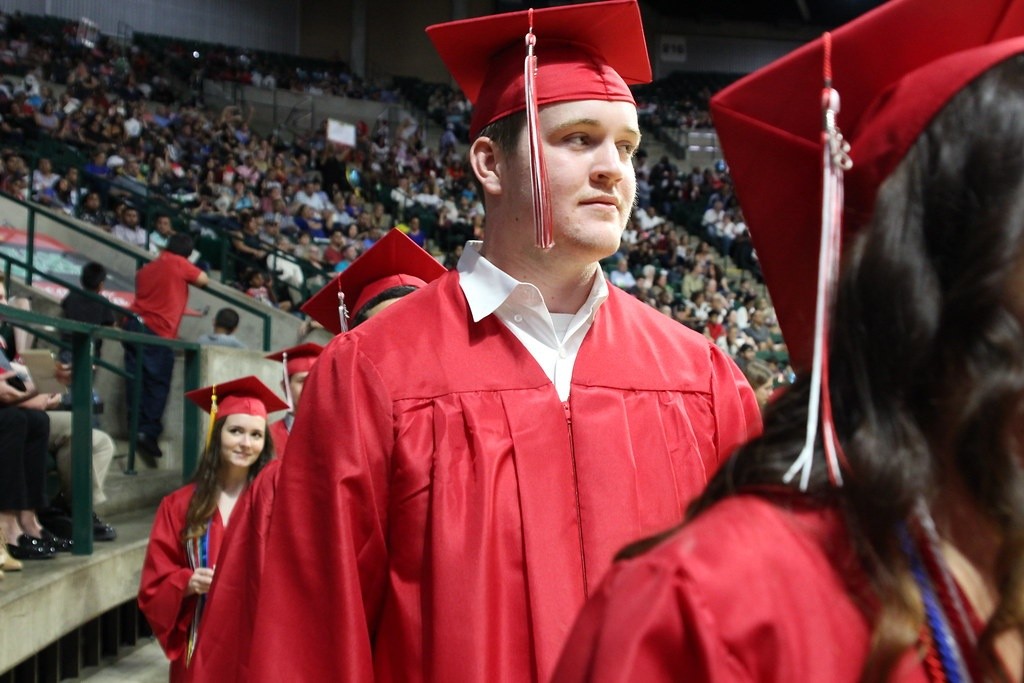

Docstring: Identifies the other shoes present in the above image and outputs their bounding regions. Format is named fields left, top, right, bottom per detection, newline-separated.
left=0, top=549, right=22, bottom=571
left=139, top=431, right=165, bottom=458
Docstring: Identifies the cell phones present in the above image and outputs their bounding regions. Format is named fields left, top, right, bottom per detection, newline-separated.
left=6, top=376, right=27, bottom=392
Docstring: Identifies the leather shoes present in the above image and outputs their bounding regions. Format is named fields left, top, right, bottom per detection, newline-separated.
left=39, top=528, right=74, bottom=551
left=95, top=513, right=114, bottom=539
left=8, top=533, right=55, bottom=558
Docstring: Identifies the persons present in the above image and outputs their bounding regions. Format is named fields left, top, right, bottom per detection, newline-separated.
left=0, top=261, right=129, bottom=580
left=122, top=232, right=209, bottom=458
left=264, top=343, right=326, bottom=463
left=553, top=1, right=1024, bottom=683
left=302, top=227, right=449, bottom=336
left=186, top=0, right=764, bottom=683
left=139, top=376, right=290, bottom=683
left=598, top=84, right=797, bottom=408
left=197, top=309, right=246, bottom=350
left=0, top=9, right=485, bottom=325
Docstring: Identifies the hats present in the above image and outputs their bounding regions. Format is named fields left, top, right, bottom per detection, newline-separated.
left=426, top=0, right=651, bottom=250
left=183, top=375, right=290, bottom=452
left=266, top=342, right=326, bottom=413
left=710, top=2, right=1022, bottom=493
left=301, top=227, right=449, bottom=336
